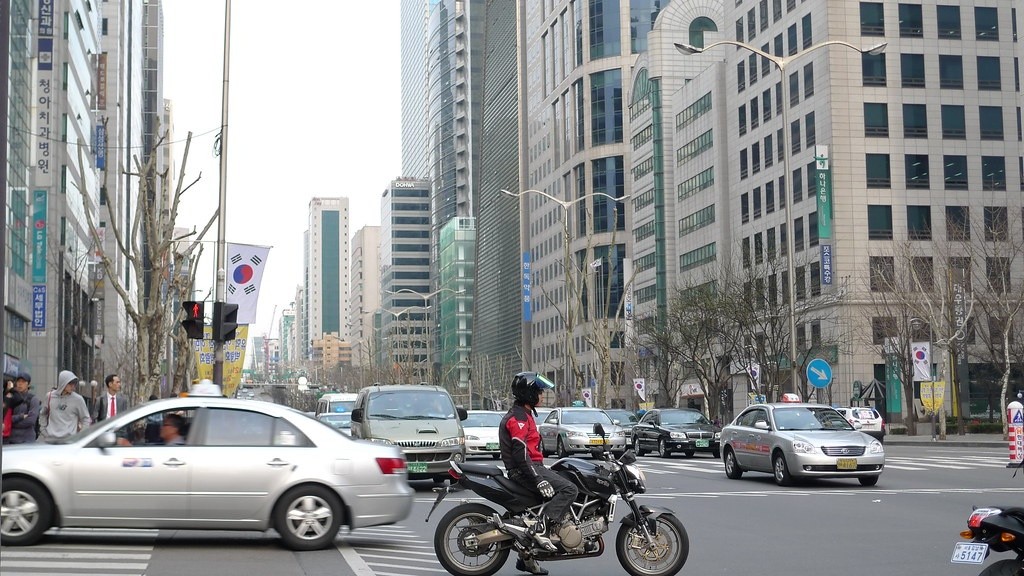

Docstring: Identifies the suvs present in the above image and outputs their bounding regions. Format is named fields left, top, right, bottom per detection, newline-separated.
left=834, top=406, right=885, bottom=445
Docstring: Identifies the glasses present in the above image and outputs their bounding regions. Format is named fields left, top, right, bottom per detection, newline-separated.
left=68, top=381, right=77, bottom=385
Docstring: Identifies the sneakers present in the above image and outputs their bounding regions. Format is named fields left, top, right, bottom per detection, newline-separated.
left=516, top=556, right=549, bottom=575
left=528, top=520, right=558, bottom=552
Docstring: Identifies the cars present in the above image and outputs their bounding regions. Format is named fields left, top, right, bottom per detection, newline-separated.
left=720, top=394, right=886, bottom=486
left=317, top=412, right=354, bottom=437
left=631, top=408, right=722, bottom=458
left=529, top=407, right=626, bottom=458
left=242, top=388, right=248, bottom=393
left=0, top=380, right=416, bottom=547
left=237, top=392, right=254, bottom=399
left=604, top=409, right=640, bottom=444
left=460, top=410, right=505, bottom=458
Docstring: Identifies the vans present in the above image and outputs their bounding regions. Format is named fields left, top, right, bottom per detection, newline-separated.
left=314, top=393, right=360, bottom=419
left=350, top=383, right=468, bottom=484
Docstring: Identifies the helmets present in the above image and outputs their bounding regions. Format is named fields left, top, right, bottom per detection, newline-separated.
left=511, top=371, right=544, bottom=406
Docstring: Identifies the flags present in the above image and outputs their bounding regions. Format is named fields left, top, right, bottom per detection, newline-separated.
left=911, top=341, right=932, bottom=381
left=581, top=387, right=592, bottom=407
left=226, top=242, right=271, bottom=324
left=632, top=378, right=645, bottom=401
left=590, top=258, right=601, bottom=267
left=748, top=365, right=759, bottom=389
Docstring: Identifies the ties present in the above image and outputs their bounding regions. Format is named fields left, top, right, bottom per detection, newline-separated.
left=111, top=397, right=115, bottom=416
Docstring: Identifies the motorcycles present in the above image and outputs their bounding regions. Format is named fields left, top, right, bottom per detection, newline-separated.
left=950, top=505, right=1024, bottom=576
left=425, top=424, right=690, bottom=576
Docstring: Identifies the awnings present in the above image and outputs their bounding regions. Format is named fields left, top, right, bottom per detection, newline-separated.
left=753, top=345, right=838, bottom=371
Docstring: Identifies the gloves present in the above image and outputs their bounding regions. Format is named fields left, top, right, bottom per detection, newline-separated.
left=537, top=480, right=555, bottom=498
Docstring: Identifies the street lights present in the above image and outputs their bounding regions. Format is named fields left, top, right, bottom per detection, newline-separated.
left=674, top=41, right=887, bottom=390
left=386, top=289, right=466, bottom=363
left=379, top=306, right=432, bottom=385
left=500, top=188, right=631, bottom=396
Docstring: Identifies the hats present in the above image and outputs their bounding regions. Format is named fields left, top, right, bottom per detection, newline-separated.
left=17, top=372, right=30, bottom=384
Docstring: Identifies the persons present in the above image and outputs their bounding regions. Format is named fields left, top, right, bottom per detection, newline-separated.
left=2, top=370, right=92, bottom=444
left=117, top=413, right=186, bottom=447
left=93, top=374, right=127, bottom=424
left=714, top=416, right=720, bottom=427
left=143, top=394, right=163, bottom=428
left=499, top=371, right=579, bottom=576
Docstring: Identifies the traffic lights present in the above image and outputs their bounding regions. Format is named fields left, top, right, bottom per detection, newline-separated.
left=182, top=301, right=204, bottom=339
left=212, top=302, right=238, bottom=343
